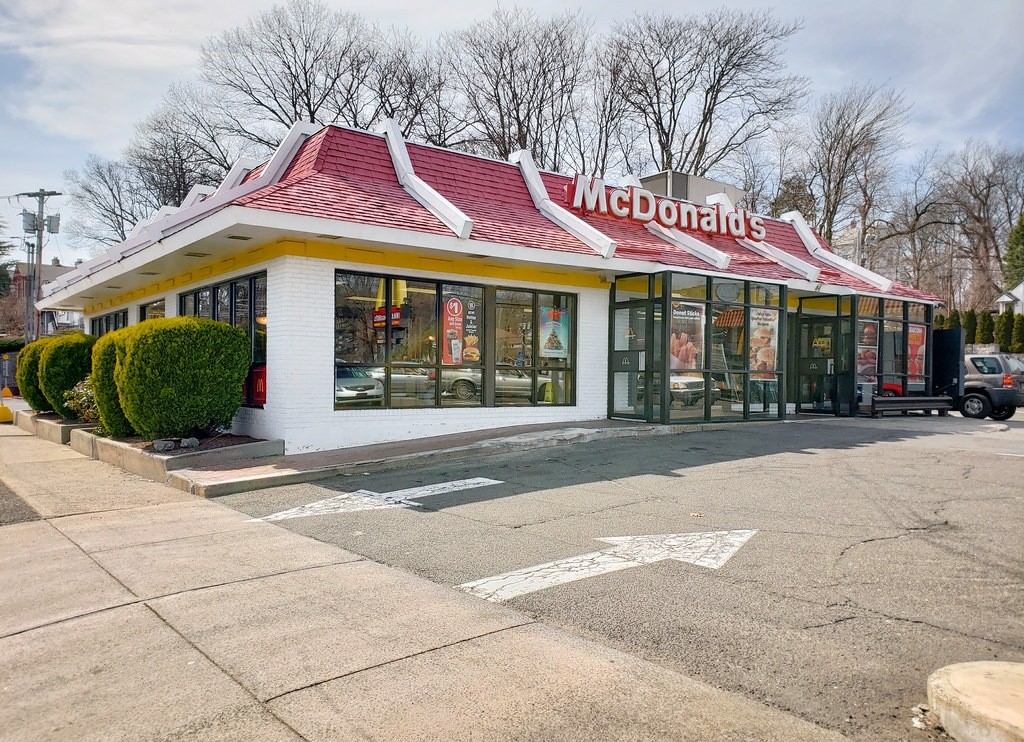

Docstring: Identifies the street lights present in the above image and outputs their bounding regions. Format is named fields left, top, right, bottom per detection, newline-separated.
left=30, top=243, right=35, bottom=343
left=522, top=314, right=530, bottom=361
left=935, top=274, right=952, bottom=319
left=25, top=241, right=31, bottom=345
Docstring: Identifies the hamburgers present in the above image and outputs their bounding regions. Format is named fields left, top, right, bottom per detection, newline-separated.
left=754, top=328, right=771, bottom=347
left=463, top=347, right=480, bottom=361
left=757, top=348, right=775, bottom=379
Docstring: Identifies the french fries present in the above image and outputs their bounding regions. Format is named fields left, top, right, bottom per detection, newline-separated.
left=466, top=335, right=477, bottom=343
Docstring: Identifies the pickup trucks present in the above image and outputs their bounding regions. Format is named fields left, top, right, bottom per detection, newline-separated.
left=424, top=362, right=564, bottom=403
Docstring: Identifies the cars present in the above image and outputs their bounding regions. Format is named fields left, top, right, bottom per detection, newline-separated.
left=335, top=358, right=384, bottom=406
left=949, top=353, right=1024, bottom=421
left=811, top=373, right=903, bottom=412
left=364, top=362, right=428, bottom=393
left=637, top=370, right=720, bottom=406
